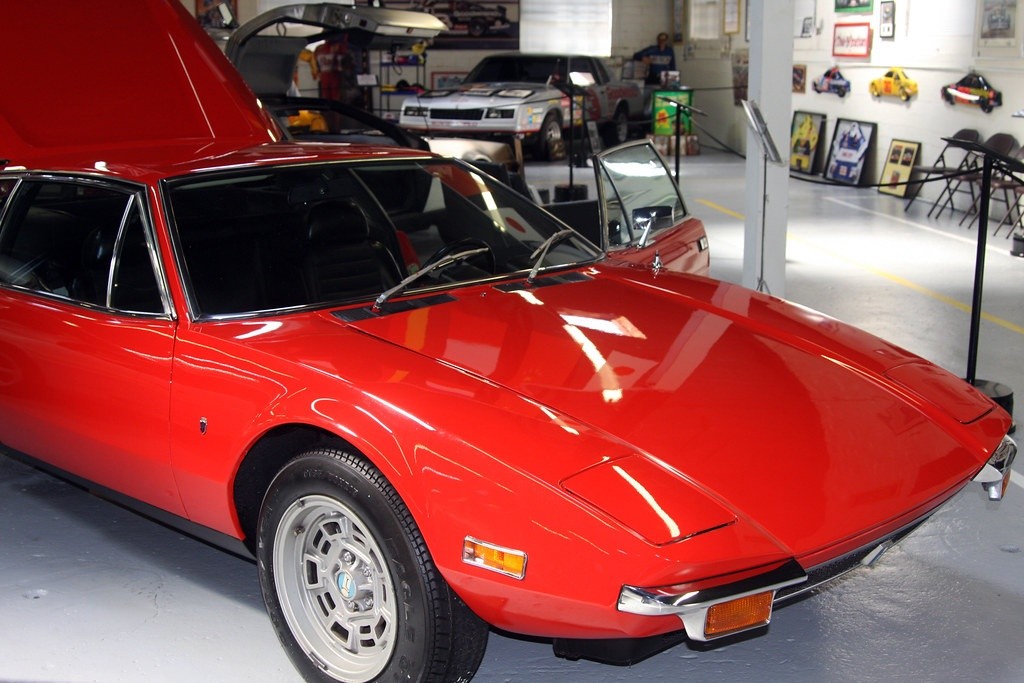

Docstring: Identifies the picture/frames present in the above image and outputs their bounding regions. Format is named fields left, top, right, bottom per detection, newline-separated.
left=880, top=1, right=894, bottom=37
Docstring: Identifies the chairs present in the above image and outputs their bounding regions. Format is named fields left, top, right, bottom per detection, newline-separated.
left=296, top=197, right=405, bottom=304
left=905, top=130, right=1024, bottom=239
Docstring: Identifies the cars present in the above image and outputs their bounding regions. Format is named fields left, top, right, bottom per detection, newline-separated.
left=400, top=51, right=645, bottom=164
left=0, top=0, right=1013, bottom=683
left=227, top=5, right=447, bottom=150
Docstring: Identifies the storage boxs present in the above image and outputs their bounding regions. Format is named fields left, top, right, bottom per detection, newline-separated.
left=645, top=133, right=699, bottom=159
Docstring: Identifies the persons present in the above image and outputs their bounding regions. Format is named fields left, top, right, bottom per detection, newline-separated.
left=634, top=33, right=675, bottom=86
left=311, top=33, right=348, bottom=100
